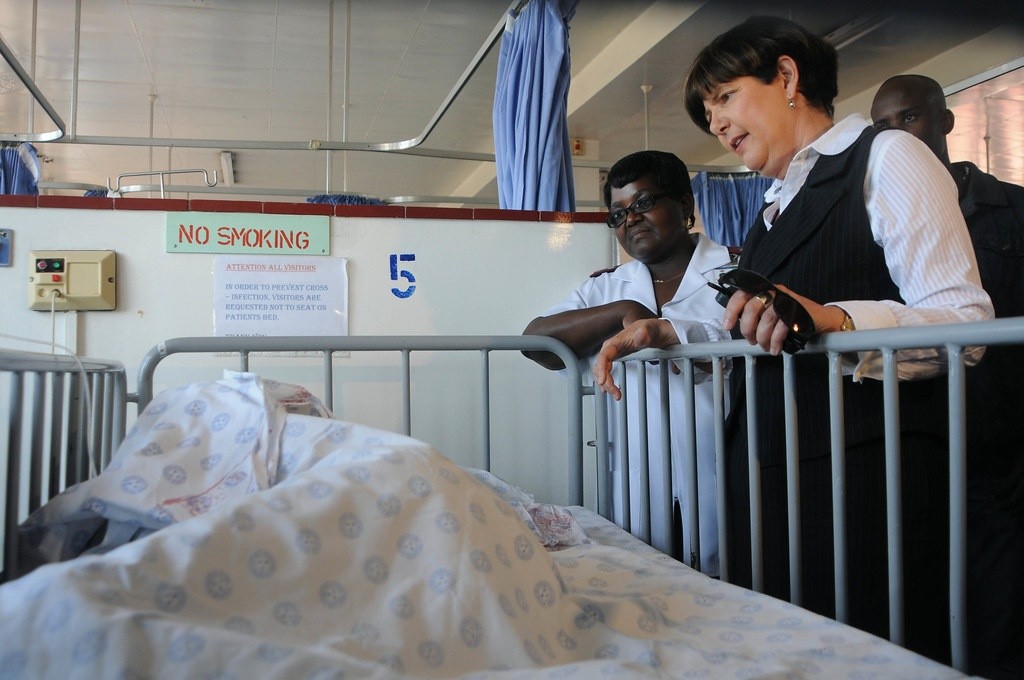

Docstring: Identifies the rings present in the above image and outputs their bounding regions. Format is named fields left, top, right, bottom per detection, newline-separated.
left=756, top=292, right=774, bottom=310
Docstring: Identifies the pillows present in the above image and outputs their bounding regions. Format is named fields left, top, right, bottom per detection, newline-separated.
left=509, top=503, right=600, bottom=548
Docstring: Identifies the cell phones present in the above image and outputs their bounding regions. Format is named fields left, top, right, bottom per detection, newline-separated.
left=715, top=286, right=801, bottom=355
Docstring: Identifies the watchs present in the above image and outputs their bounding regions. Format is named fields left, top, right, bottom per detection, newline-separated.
left=841, top=309, right=855, bottom=332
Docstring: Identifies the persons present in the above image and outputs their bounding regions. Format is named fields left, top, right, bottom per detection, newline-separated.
left=522, top=151, right=741, bottom=581
left=595, top=14, right=995, bottom=634
left=870, top=73, right=1024, bottom=679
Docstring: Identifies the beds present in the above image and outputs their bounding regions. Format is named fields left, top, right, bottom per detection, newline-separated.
left=0, top=316, right=1024, bottom=680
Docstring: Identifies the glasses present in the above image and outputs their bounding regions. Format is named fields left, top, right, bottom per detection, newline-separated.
left=706, top=269, right=816, bottom=355
left=605, top=189, right=679, bottom=229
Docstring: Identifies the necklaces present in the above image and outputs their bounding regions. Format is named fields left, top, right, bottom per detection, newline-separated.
left=653, top=272, right=684, bottom=283
